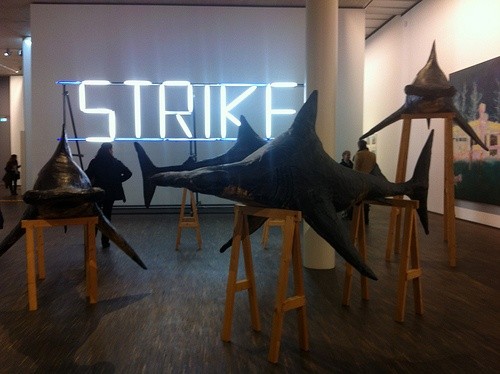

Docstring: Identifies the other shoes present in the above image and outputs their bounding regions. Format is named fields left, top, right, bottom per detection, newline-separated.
left=342, top=215, right=353, bottom=221
left=103, top=242, right=111, bottom=249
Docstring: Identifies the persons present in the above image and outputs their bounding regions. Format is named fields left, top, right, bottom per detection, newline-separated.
left=4, top=154, right=21, bottom=196
left=352, top=139, right=376, bottom=224
left=84, top=142, right=133, bottom=249
left=338, top=150, right=354, bottom=222
